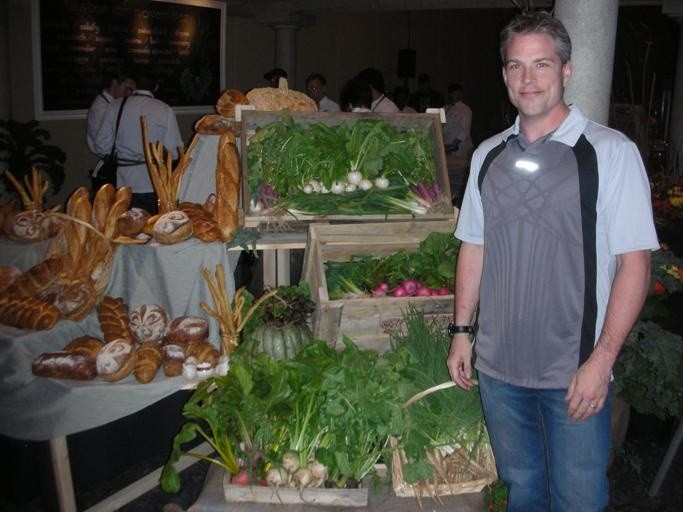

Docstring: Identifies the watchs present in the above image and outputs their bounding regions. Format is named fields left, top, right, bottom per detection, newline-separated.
left=445, top=321, right=477, bottom=339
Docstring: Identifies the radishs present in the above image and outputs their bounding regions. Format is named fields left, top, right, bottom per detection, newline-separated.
left=301, top=170, right=390, bottom=194
left=267, top=451, right=329, bottom=489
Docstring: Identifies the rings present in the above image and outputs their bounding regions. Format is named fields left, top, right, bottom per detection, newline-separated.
left=589, top=403, right=599, bottom=409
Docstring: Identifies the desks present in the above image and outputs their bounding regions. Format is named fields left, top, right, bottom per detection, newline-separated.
left=226, top=228, right=307, bottom=293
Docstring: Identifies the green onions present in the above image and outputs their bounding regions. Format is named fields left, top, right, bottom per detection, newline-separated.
left=365, top=186, right=431, bottom=215
left=394, top=302, right=495, bottom=482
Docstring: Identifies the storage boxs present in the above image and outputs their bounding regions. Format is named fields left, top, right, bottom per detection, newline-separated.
left=303, top=215, right=459, bottom=353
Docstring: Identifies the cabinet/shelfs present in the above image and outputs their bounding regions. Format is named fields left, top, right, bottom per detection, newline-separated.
left=0, top=233, right=236, bottom=511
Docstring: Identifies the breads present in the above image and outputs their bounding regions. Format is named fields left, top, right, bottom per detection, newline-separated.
left=0, top=87, right=317, bottom=384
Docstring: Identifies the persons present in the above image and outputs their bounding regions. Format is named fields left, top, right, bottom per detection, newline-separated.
left=353, top=69, right=402, bottom=113
left=339, top=78, right=374, bottom=113
left=92, top=66, right=187, bottom=214
left=438, top=82, right=473, bottom=207
left=406, top=72, right=444, bottom=114
left=261, top=68, right=289, bottom=94
left=446, top=1, right=662, bottom=510
left=84, top=63, right=134, bottom=204
left=305, top=75, right=342, bottom=114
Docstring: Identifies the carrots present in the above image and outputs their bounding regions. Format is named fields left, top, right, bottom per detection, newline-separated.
left=372, top=280, right=450, bottom=297
left=230, top=447, right=270, bottom=487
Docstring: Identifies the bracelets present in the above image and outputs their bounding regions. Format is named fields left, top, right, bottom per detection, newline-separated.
left=448, top=144, right=455, bottom=150
